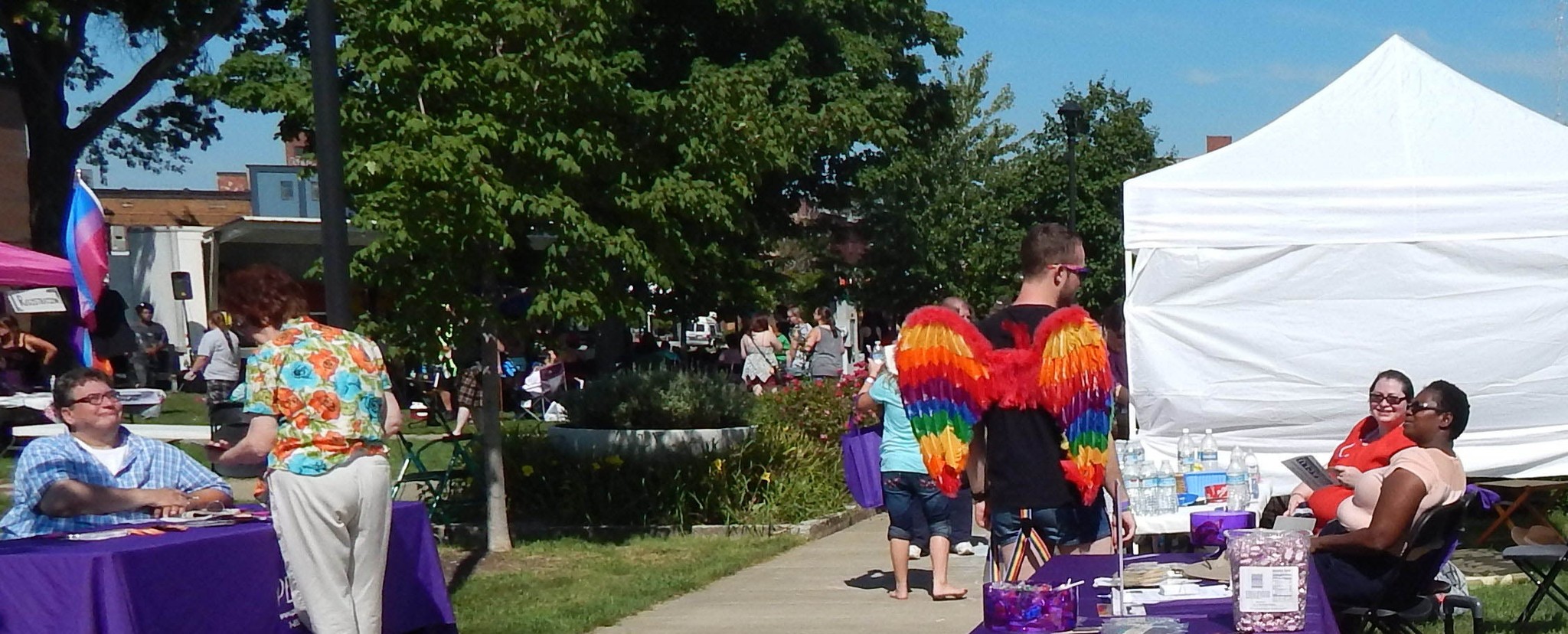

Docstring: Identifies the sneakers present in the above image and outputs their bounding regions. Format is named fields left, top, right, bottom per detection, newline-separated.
left=908, top=544, right=922, bottom=558
left=955, top=542, right=975, bottom=555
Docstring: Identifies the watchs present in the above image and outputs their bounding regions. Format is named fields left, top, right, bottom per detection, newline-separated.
left=866, top=377, right=874, bottom=384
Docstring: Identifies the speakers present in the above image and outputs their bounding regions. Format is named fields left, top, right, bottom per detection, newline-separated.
left=171, top=271, right=193, bottom=300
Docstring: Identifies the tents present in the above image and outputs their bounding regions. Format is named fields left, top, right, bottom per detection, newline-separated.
left=0, top=245, right=86, bottom=371
left=1122, top=35, right=1567, bottom=499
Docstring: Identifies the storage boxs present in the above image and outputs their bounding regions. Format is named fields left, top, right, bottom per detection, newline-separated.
left=1183, top=472, right=1228, bottom=498
left=1224, top=529, right=1313, bottom=634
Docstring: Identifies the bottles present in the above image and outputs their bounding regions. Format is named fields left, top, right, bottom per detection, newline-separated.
left=1226, top=445, right=1259, bottom=513
left=873, top=341, right=887, bottom=380
left=1178, top=427, right=1195, bottom=473
left=50, top=374, right=56, bottom=391
left=1115, top=437, right=1178, bottom=516
left=1198, top=428, right=1218, bottom=471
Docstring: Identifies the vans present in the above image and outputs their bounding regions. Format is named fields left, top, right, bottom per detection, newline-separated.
left=685, top=316, right=720, bottom=349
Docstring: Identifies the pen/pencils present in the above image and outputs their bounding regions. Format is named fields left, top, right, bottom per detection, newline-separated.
left=1123, top=553, right=1160, bottom=561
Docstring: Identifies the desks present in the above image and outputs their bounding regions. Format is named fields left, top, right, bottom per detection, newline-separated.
left=0, top=389, right=220, bottom=456
left=0, top=501, right=458, bottom=634
left=1106, top=483, right=1273, bottom=553
left=1475, top=479, right=1568, bottom=547
left=971, top=553, right=1340, bottom=634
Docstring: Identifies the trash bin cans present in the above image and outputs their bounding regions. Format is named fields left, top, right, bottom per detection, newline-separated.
left=208, top=401, right=267, bottom=479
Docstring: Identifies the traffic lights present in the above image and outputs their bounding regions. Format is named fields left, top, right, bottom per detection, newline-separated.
left=829, top=271, right=848, bottom=294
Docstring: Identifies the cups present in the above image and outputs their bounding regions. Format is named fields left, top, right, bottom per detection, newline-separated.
left=1224, top=528, right=1313, bottom=633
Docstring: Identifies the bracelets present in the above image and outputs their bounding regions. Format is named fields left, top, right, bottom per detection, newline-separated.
left=189, top=496, right=201, bottom=510
left=970, top=487, right=985, bottom=503
left=868, top=376, right=876, bottom=381
left=1113, top=499, right=1132, bottom=512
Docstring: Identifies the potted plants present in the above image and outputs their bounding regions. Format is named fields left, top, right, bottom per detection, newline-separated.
left=549, top=357, right=759, bottom=466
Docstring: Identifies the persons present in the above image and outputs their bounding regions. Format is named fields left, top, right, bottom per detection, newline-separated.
left=206, top=265, right=403, bottom=634
left=719, top=219, right=1470, bottom=634
left=1, top=276, right=676, bottom=543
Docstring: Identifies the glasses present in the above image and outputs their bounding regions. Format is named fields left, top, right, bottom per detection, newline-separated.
left=72, top=391, right=121, bottom=405
left=1371, top=392, right=1405, bottom=405
left=1406, top=401, right=1450, bottom=414
left=1048, top=262, right=1089, bottom=283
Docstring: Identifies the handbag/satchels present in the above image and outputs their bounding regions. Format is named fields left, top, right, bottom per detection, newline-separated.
left=842, top=397, right=886, bottom=509
left=775, top=367, right=789, bottom=383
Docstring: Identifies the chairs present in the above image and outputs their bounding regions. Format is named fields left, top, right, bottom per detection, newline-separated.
left=391, top=408, right=484, bottom=525
left=513, top=361, right=568, bottom=421
left=1329, top=493, right=1474, bottom=634
left=1503, top=545, right=1568, bottom=634
left=209, top=403, right=270, bottom=479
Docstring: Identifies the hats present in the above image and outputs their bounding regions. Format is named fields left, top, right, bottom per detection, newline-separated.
left=135, top=302, right=153, bottom=311
